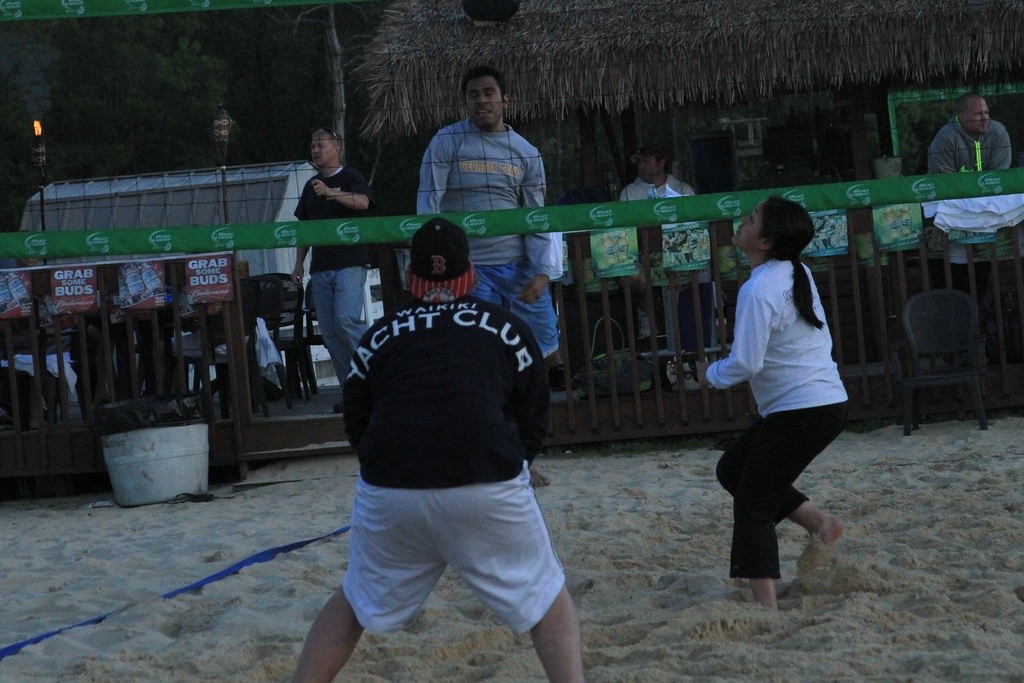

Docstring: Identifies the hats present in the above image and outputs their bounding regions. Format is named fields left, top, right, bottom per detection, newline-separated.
left=630, top=144, right=668, bottom=164
left=408, top=217, right=474, bottom=303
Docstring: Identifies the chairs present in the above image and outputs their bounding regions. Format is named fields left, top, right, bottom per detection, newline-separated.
left=172, top=273, right=326, bottom=417
left=896, top=288, right=991, bottom=436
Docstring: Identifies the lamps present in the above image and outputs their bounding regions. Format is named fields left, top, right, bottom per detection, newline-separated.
left=722, top=116, right=767, bottom=158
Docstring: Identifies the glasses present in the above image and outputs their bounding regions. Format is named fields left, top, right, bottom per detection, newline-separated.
left=310, top=126, right=338, bottom=139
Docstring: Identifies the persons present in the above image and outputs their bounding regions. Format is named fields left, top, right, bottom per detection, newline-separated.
left=414, top=64, right=562, bottom=486
left=927, top=92, right=1011, bottom=174
left=698, top=197, right=849, bottom=610
left=619, top=143, right=694, bottom=200
left=291, top=128, right=378, bottom=414
left=294, top=218, right=583, bottom=683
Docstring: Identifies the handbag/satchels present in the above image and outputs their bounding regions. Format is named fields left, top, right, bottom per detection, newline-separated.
left=562, top=317, right=669, bottom=398
left=666, top=349, right=714, bottom=392
